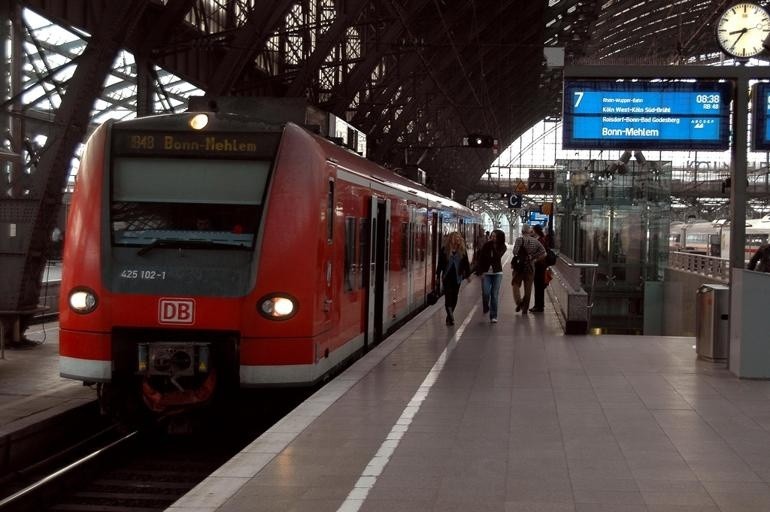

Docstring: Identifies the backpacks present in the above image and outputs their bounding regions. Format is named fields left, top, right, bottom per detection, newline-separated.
left=511, top=246, right=533, bottom=274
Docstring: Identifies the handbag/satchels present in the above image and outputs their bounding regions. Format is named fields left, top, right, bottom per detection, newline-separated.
left=542, top=249, right=557, bottom=265
left=427, top=285, right=441, bottom=305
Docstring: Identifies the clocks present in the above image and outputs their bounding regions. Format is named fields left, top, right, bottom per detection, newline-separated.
left=714, top=2, right=770, bottom=59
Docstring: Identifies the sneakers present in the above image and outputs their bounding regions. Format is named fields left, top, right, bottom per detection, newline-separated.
left=481, top=303, right=544, bottom=324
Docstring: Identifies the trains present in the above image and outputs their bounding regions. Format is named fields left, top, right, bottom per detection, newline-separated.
left=58, top=96, right=492, bottom=429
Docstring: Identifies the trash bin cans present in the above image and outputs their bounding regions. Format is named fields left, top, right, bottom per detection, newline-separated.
left=696, top=283, right=729, bottom=364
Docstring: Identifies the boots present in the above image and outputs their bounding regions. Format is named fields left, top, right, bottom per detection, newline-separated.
left=444, top=306, right=455, bottom=326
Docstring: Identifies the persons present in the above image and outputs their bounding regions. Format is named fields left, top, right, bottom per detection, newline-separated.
left=436, top=223, right=555, bottom=326
left=748, top=243, right=770, bottom=272
